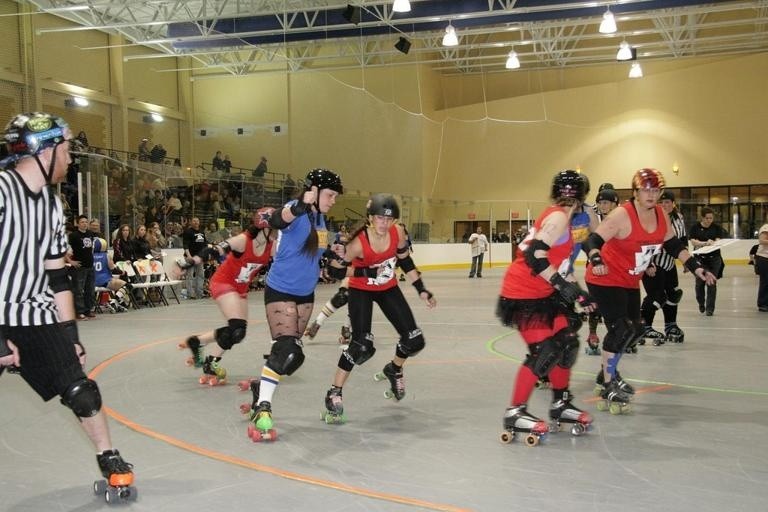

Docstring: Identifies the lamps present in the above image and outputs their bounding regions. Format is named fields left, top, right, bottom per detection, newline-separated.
left=628, top=61, right=643, bottom=78
left=599, top=3, right=617, bottom=34
left=392, top=0, right=411, bottom=13
left=65, top=98, right=78, bottom=108
left=395, top=37, right=411, bottom=54
left=442, top=19, right=458, bottom=46
left=143, top=115, right=155, bottom=122
left=617, top=35, right=632, bottom=60
left=618, top=48, right=638, bottom=63
left=343, top=5, right=359, bottom=25
left=505, top=44, right=521, bottom=69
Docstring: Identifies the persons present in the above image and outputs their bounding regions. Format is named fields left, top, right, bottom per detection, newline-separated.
left=640, top=189, right=687, bottom=339
left=60, top=132, right=354, bottom=321
left=689, top=207, right=727, bottom=317
left=587, top=183, right=615, bottom=350
left=398, top=222, right=420, bottom=281
left=303, top=198, right=373, bottom=352
left=563, top=173, right=590, bottom=280
left=754, top=216, right=768, bottom=311
left=495, top=169, right=595, bottom=434
left=248, top=169, right=343, bottom=430
left=176, top=207, right=279, bottom=380
left=325, top=193, right=437, bottom=413
left=467, top=226, right=488, bottom=278
left=512, top=229, right=528, bottom=246
left=0, top=111, right=135, bottom=489
left=596, top=189, right=619, bottom=217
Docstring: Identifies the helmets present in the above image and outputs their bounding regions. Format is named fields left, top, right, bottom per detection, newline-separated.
left=304, top=168, right=344, bottom=195
left=3, top=111, right=74, bottom=156
left=92, top=238, right=108, bottom=252
left=550, top=165, right=677, bottom=206
left=252, top=207, right=277, bottom=230
left=367, top=192, right=400, bottom=219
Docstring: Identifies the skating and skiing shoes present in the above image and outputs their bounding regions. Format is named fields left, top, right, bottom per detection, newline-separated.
left=497, top=323, right=686, bottom=448
left=93, top=449, right=137, bottom=505
left=103, top=298, right=118, bottom=314
left=177, top=324, right=406, bottom=443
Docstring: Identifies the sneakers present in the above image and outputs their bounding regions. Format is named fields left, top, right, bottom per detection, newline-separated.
left=706, top=312, right=713, bottom=317
left=75, top=313, right=89, bottom=321
left=698, top=304, right=706, bottom=314
left=118, top=301, right=128, bottom=312
left=86, top=311, right=97, bottom=317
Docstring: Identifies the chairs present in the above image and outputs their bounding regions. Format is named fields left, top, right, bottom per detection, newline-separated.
left=133, top=261, right=167, bottom=306
left=111, top=264, right=137, bottom=310
left=151, top=260, right=181, bottom=306
left=93, top=286, right=111, bottom=314
left=116, top=261, right=156, bottom=309
